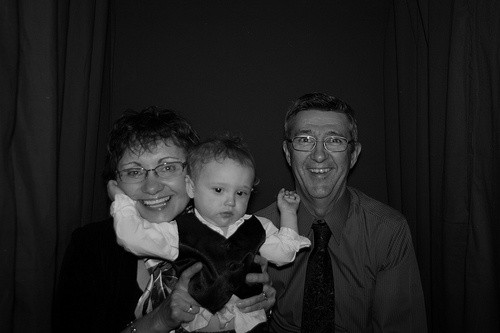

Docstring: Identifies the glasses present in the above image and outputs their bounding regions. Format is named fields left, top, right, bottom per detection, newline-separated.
left=115, top=161, right=190, bottom=184
left=286, top=135, right=357, bottom=154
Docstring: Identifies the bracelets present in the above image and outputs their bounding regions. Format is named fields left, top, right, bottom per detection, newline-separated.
left=129, top=321, right=136, bottom=333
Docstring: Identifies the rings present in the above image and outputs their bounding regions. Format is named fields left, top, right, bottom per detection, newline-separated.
left=187, top=303, right=193, bottom=313
left=263, top=292, right=267, bottom=299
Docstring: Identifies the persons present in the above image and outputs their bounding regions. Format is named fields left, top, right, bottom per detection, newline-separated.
left=251, top=92, right=428, bottom=333
left=106, top=134, right=311, bottom=333
left=52, top=107, right=276, bottom=333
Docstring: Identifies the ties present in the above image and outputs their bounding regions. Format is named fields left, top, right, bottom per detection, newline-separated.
left=301, top=220, right=337, bottom=333
